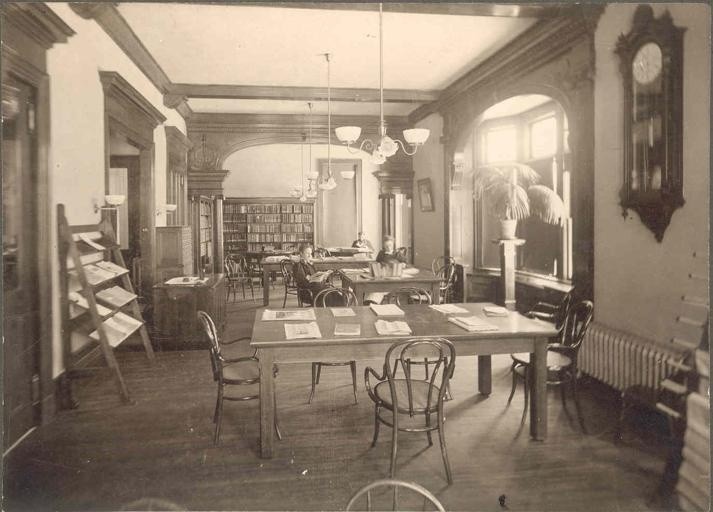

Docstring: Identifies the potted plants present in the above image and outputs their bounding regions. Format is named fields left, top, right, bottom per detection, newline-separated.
left=465, top=161, right=570, bottom=239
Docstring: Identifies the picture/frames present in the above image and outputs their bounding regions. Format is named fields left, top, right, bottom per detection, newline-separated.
left=416, top=178, right=434, bottom=212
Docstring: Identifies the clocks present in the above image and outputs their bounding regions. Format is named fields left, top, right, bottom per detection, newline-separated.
left=611, top=3, right=689, bottom=243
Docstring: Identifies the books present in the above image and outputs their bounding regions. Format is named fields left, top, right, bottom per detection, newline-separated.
left=334, top=323, right=361, bottom=335
left=369, top=302, right=404, bottom=316
left=369, top=260, right=401, bottom=278
left=223, top=204, right=314, bottom=253
left=309, top=269, right=333, bottom=283
left=67, top=233, right=143, bottom=348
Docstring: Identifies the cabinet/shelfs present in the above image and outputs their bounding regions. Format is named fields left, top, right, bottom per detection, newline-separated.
left=222, top=203, right=246, bottom=249
left=55, top=202, right=155, bottom=408
left=281, top=203, right=313, bottom=250
left=152, top=273, right=223, bottom=349
left=246, top=203, right=281, bottom=249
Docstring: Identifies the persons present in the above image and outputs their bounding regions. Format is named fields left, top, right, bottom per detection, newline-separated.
left=352, top=232, right=377, bottom=259
left=376, top=235, right=407, bottom=266
left=293, top=242, right=344, bottom=307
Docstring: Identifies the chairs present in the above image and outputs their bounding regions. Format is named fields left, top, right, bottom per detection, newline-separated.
left=613, top=347, right=693, bottom=442
left=501, top=299, right=595, bottom=440
left=117, top=496, right=188, bottom=512
left=527, top=292, right=573, bottom=343
left=363, top=337, right=457, bottom=490
left=307, top=286, right=362, bottom=405
left=223, top=245, right=458, bottom=306
left=385, top=285, right=449, bottom=381
left=343, top=478, right=446, bottom=512
left=194, top=310, right=283, bottom=447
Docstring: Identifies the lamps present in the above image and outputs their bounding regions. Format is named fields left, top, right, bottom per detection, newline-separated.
left=286, top=53, right=354, bottom=203
left=334, top=1, right=430, bottom=165
left=94, top=195, right=125, bottom=214
left=153, top=203, right=177, bottom=216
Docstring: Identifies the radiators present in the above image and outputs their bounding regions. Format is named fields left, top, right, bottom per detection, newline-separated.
left=575, top=322, right=690, bottom=397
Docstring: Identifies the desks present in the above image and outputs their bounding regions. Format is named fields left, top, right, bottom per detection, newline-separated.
left=247, top=300, right=559, bottom=459
